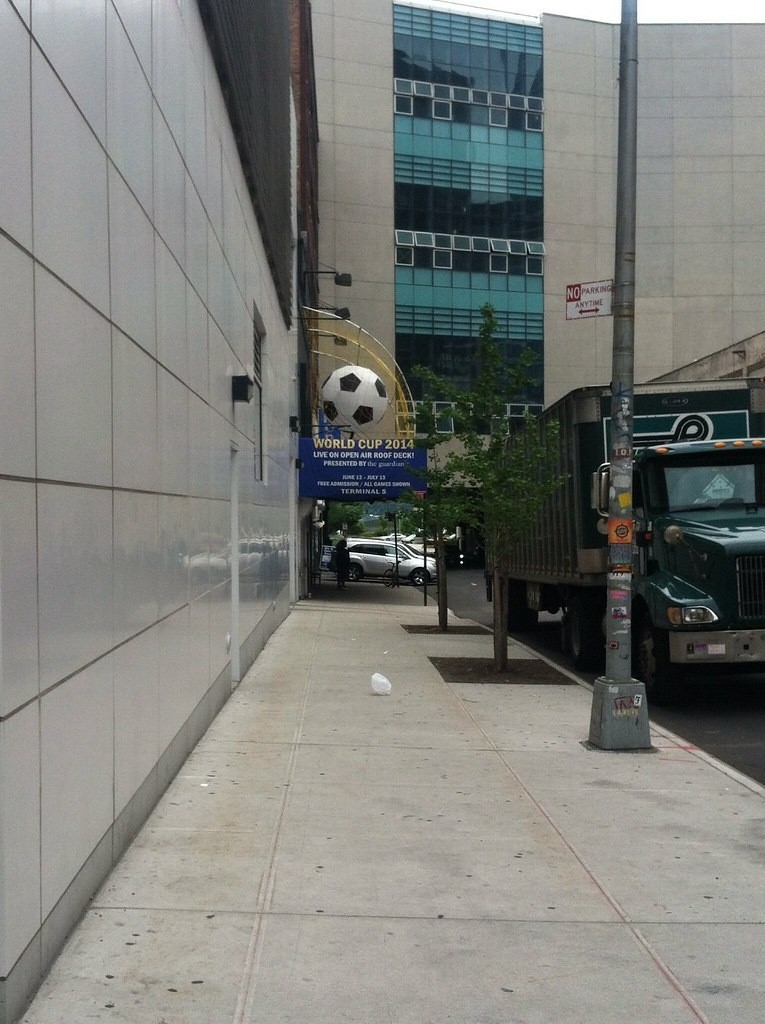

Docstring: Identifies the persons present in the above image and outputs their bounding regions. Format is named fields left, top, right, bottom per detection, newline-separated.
left=336, top=539, right=350, bottom=590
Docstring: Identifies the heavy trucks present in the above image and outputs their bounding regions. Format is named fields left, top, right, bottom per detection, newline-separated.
left=485, top=375, right=765, bottom=705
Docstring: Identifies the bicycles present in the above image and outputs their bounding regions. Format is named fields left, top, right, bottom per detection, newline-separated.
left=382, top=561, right=401, bottom=587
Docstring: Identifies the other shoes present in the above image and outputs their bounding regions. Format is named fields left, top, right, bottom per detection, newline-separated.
left=337, top=585, right=349, bottom=591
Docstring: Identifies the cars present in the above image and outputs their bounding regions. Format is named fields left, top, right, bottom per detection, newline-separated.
left=346, top=542, right=438, bottom=586
left=378, top=541, right=436, bottom=566
left=384, top=533, right=406, bottom=542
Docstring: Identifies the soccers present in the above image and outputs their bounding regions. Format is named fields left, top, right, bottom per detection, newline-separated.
left=320, top=366, right=389, bottom=431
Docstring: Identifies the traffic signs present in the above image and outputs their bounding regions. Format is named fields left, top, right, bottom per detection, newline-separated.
left=565, top=279, right=614, bottom=321
